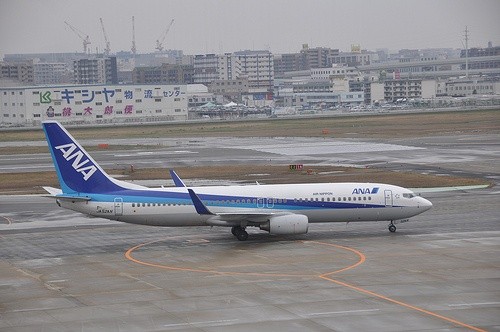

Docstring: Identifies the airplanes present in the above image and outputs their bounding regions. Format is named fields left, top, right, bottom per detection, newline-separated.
left=38, top=121, right=433, bottom=241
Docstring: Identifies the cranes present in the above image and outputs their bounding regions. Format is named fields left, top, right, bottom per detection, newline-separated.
left=129, top=14, right=138, bottom=53
left=99, top=17, right=112, bottom=56
left=156, top=20, right=175, bottom=51
left=62, top=21, right=92, bottom=58
